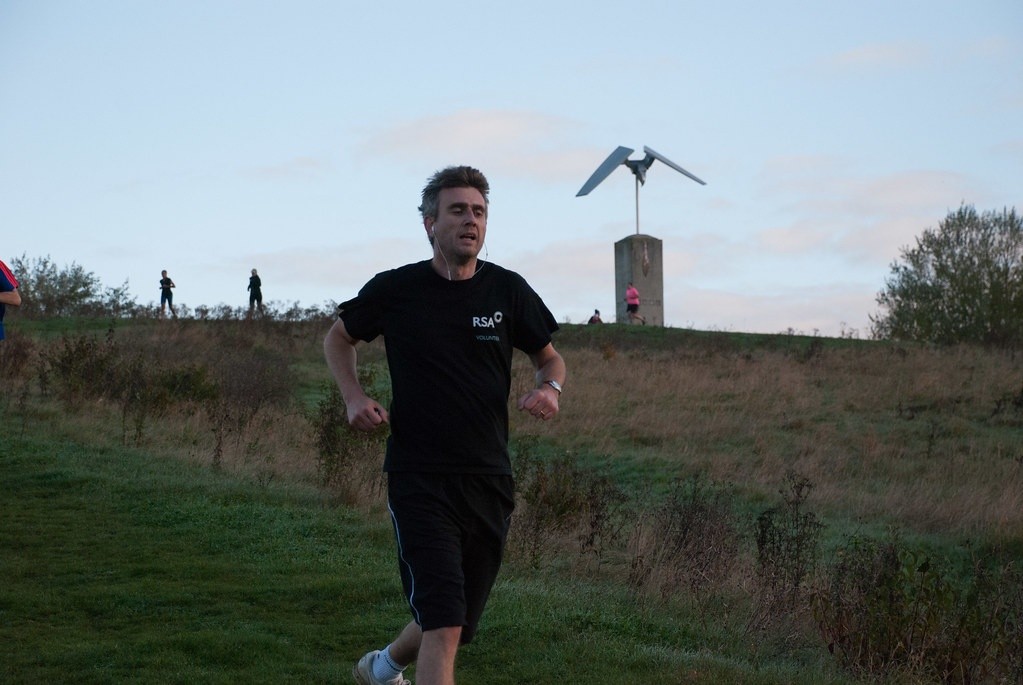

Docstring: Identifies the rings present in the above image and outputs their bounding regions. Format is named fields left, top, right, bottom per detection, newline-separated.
left=540, top=410, right=545, bottom=416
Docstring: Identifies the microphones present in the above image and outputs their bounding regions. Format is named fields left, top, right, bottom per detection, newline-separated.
left=447, top=270, right=451, bottom=280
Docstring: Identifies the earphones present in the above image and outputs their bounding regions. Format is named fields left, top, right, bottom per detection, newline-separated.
left=431, top=225, right=435, bottom=232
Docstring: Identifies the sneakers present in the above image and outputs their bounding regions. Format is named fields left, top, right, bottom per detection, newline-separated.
left=352, top=649, right=403, bottom=685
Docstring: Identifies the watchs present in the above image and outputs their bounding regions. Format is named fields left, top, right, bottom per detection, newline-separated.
left=543, top=379, right=562, bottom=395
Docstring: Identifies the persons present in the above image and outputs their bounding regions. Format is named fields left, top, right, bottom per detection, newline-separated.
left=586, top=310, right=603, bottom=325
left=158, top=270, right=179, bottom=319
left=247, top=268, right=264, bottom=321
left=0, top=260, right=22, bottom=341
left=325, top=164, right=567, bottom=684
left=623, top=282, right=646, bottom=325
